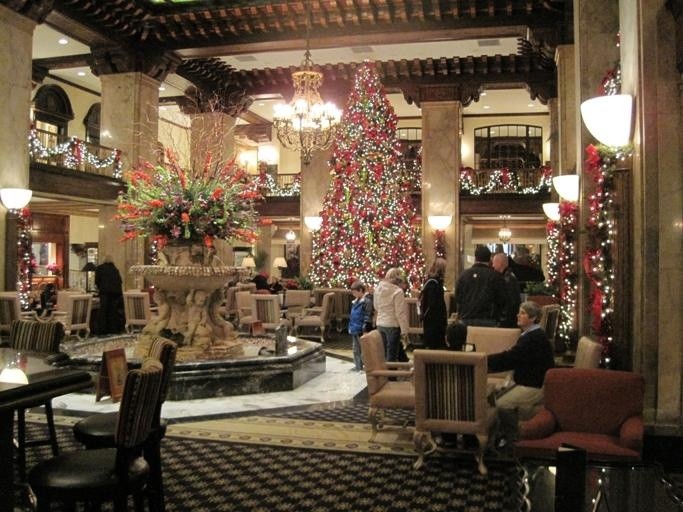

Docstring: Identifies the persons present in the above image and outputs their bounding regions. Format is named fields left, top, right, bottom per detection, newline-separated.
left=184, top=289, right=212, bottom=347
left=141, top=291, right=171, bottom=337
left=344, top=280, right=374, bottom=374
left=251, top=270, right=271, bottom=295
left=94, top=252, right=127, bottom=333
left=39, top=282, right=56, bottom=308
left=268, top=276, right=283, bottom=294
left=370, top=245, right=555, bottom=453
left=209, top=289, right=234, bottom=341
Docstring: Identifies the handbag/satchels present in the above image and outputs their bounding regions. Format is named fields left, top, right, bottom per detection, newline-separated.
left=415, top=292, right=427, bottom=322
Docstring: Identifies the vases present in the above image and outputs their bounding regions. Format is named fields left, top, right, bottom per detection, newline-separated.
left=160, top=241, right=214, bottom=266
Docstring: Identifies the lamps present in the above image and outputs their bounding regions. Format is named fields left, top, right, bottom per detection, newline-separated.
left=271, top=8, right=344, bottom=169
left=550, top=172, right=578, bottom=206
left=240, top=255, right=256, bottom=277
left=426, top=214, right=453, bottom=230
left=1, top=187, right=33, bottom=213
left=498, top=217, right=513, bottom=245
left=578, top=93, right=634, bottom=151
left=303, top=215, right=323, bottom=230
left=542, top=202, right=562, bottom=222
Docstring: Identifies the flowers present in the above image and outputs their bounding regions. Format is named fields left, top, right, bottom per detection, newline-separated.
left=100, top=87, right=277, bottom=248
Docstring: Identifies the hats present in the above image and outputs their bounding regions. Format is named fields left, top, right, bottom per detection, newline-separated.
left=474, top=246, right=489, bottom=262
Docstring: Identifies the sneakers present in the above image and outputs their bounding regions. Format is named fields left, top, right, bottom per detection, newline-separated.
left=350, top=367, right=362, bottom=373
left=358, top=367, right=366, bottom=373
